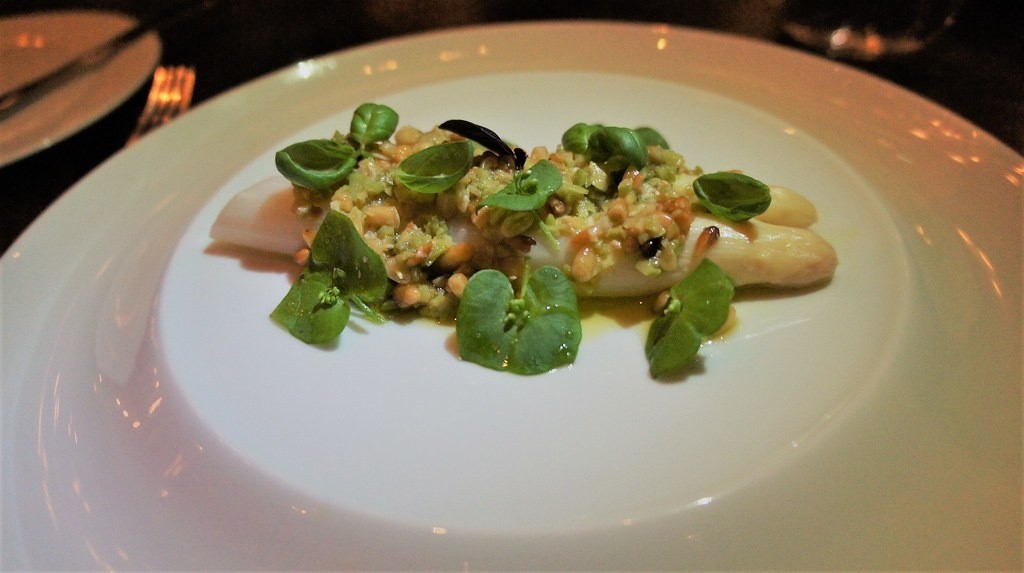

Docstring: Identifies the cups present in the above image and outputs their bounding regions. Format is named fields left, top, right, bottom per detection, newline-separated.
left=779, top=1, right=959, bottom=56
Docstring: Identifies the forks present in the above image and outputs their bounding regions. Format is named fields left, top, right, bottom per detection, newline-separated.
left=121, top=65, right=194, bottom=151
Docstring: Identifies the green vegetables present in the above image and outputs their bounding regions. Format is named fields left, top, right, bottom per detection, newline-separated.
left=272, top=104, right=773, bottom=379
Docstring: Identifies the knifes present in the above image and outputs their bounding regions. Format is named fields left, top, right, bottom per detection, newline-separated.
left=1, top=3, right=203, bottom=118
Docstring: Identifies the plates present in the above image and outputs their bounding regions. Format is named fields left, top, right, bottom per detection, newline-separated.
left=0, top=15, right=160, bottom=169
left=0, top=21, right=1021, bottom=573
